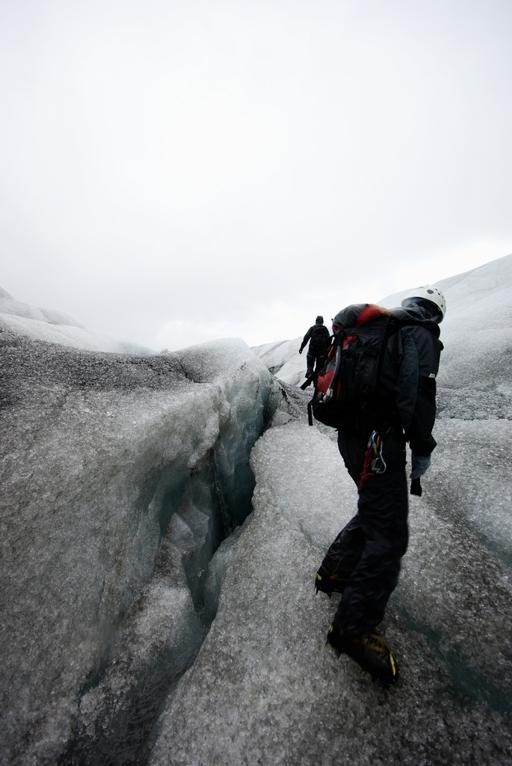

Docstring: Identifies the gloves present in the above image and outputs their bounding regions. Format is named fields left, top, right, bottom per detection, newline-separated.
left=407, top=457, right=432, bottom=481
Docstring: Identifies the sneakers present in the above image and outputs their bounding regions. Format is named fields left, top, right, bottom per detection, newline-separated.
left=326, top=622, right=402, bottom=688
left=313, top=567, right=344, bottom=598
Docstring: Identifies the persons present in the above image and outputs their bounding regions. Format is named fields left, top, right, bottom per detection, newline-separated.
left=298, top=316, right=330, bottom=384
left=312, top=295, right=446, bottom=686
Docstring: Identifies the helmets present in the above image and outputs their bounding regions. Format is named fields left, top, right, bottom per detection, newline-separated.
left=399, top=285, right=448, bottom=322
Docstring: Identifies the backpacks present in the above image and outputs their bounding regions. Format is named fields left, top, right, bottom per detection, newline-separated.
left=310, top=303, right=410, bottom=431
